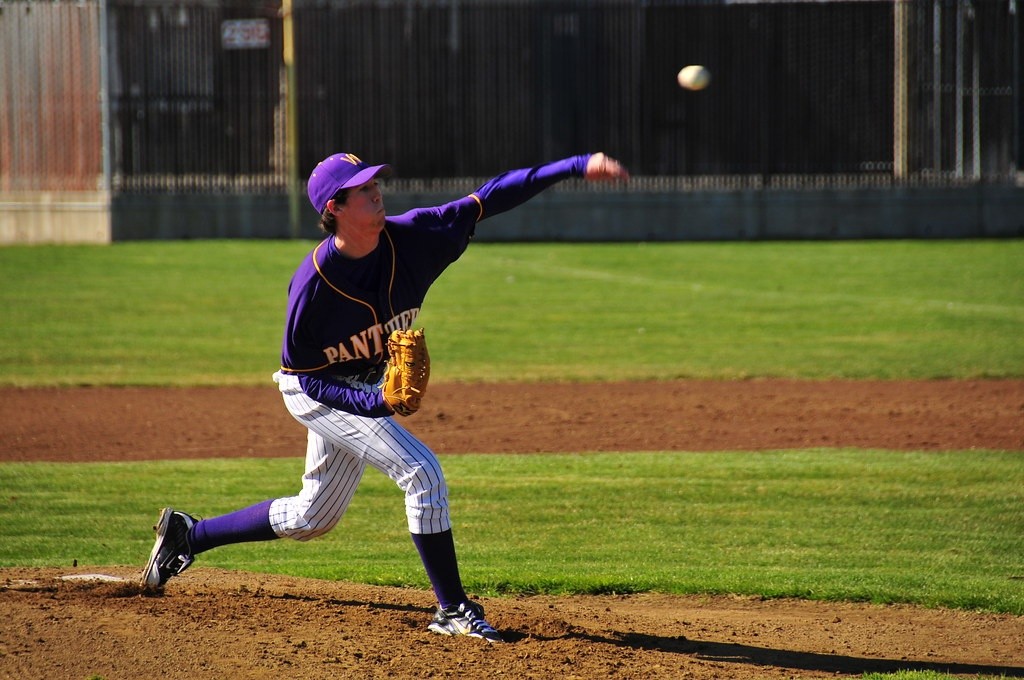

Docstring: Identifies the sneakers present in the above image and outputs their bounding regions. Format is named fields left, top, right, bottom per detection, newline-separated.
left=427, top=600, right=506, bottom=643
left=142, top=507, right=203, bottom=588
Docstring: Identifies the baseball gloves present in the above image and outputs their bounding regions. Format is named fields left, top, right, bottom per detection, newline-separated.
left=380, top=324, right=432, bottom=418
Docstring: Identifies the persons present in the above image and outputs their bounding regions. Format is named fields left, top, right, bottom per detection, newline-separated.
left=137, top=150, right=627, bottom=643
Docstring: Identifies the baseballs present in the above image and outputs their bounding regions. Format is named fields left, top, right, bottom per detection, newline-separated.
left=677, top=64, right=710, bottom=90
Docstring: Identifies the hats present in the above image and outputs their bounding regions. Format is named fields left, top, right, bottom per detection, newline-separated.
left=307, top=152, right=393, bottom=214
